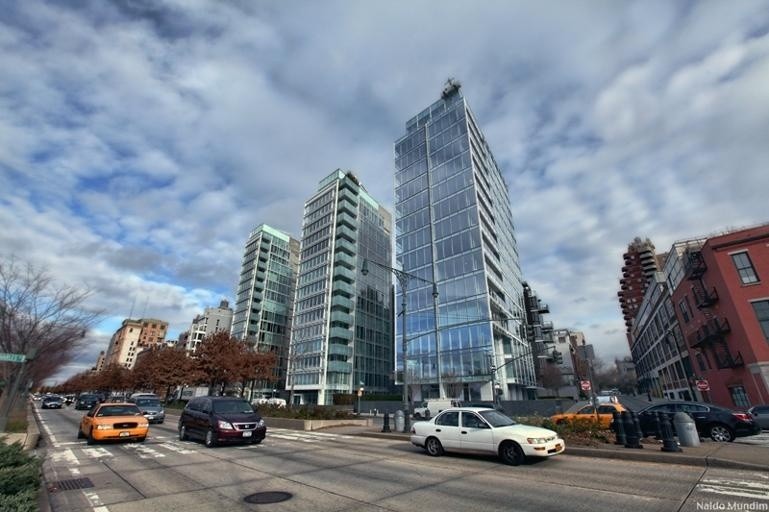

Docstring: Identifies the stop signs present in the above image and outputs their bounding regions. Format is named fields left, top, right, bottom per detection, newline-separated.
left=696, top=380, right=710, bottom=391
left=581, top=381, right=591, bottom=391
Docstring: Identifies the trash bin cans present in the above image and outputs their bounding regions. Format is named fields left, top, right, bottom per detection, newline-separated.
left=394, top=410, right=406, bottom=432
left=674, top=412, right=700, bottom=447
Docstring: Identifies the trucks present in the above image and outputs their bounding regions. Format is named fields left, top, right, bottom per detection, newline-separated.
left=412, top=397, right=460, bottom=420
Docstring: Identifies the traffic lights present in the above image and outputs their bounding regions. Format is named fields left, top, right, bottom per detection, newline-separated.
left=554, top=351, right=563, bottom=364
left=526, top=324, right=535, bottom=342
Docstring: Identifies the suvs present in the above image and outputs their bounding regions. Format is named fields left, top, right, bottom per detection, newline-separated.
left=95, top=389, right=112, bottom=403
left=130, top=392, right=158, bottom=400
left=747, top=404, right=769, bottom=430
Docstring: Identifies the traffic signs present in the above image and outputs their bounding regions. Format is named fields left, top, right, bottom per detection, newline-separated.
left=0, top=353, right=26, bottom=362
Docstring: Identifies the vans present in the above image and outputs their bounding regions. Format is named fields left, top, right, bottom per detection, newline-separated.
left=179, top=396, right=266, bottom=449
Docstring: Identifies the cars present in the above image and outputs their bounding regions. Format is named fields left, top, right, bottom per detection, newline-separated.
left=610, top=400, right=761, bottom=443
left=410, top=407, right=565, bottom=466
left=105, top=396, right=126, bottom=403
left=31, top=391, right=75, bottom=403
left=80, top=403, right=149, bottom=443
left=76, top=394, right=101, bottom=411
left=594, top=388, right=622, bottom=403
left=551, top=403, right=632, bottom=430
left=43, top=396, right=62, bottom=409
left=127, top=396, right=165, bottom=424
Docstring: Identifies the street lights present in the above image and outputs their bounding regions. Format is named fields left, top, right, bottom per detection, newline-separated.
left=359, top=257, right=439, bottom=427
left=667, top=329, right=698, bottom=401
